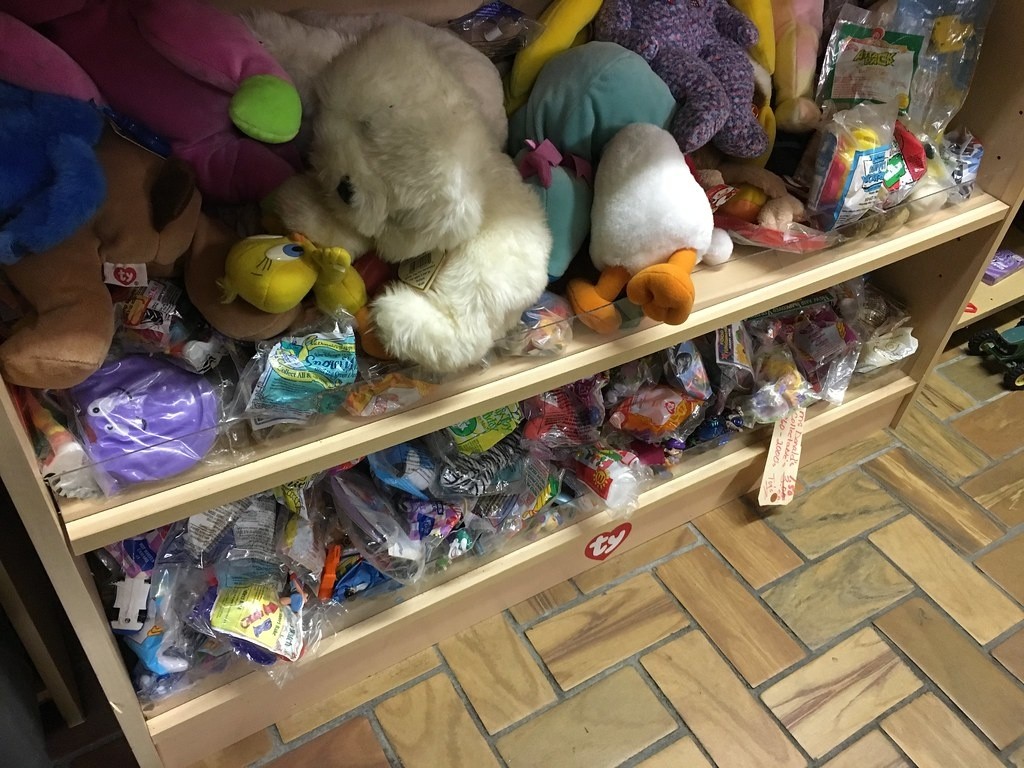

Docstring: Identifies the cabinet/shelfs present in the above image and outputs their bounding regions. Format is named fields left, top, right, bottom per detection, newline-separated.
left=1, top=1, right=1024, bottom=768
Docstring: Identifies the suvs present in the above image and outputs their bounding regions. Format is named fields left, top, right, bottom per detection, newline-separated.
left=967, top=318, right=1024, bottom=391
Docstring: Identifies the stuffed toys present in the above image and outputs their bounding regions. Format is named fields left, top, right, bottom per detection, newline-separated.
left=0, top=0, right=825, bottom=389
